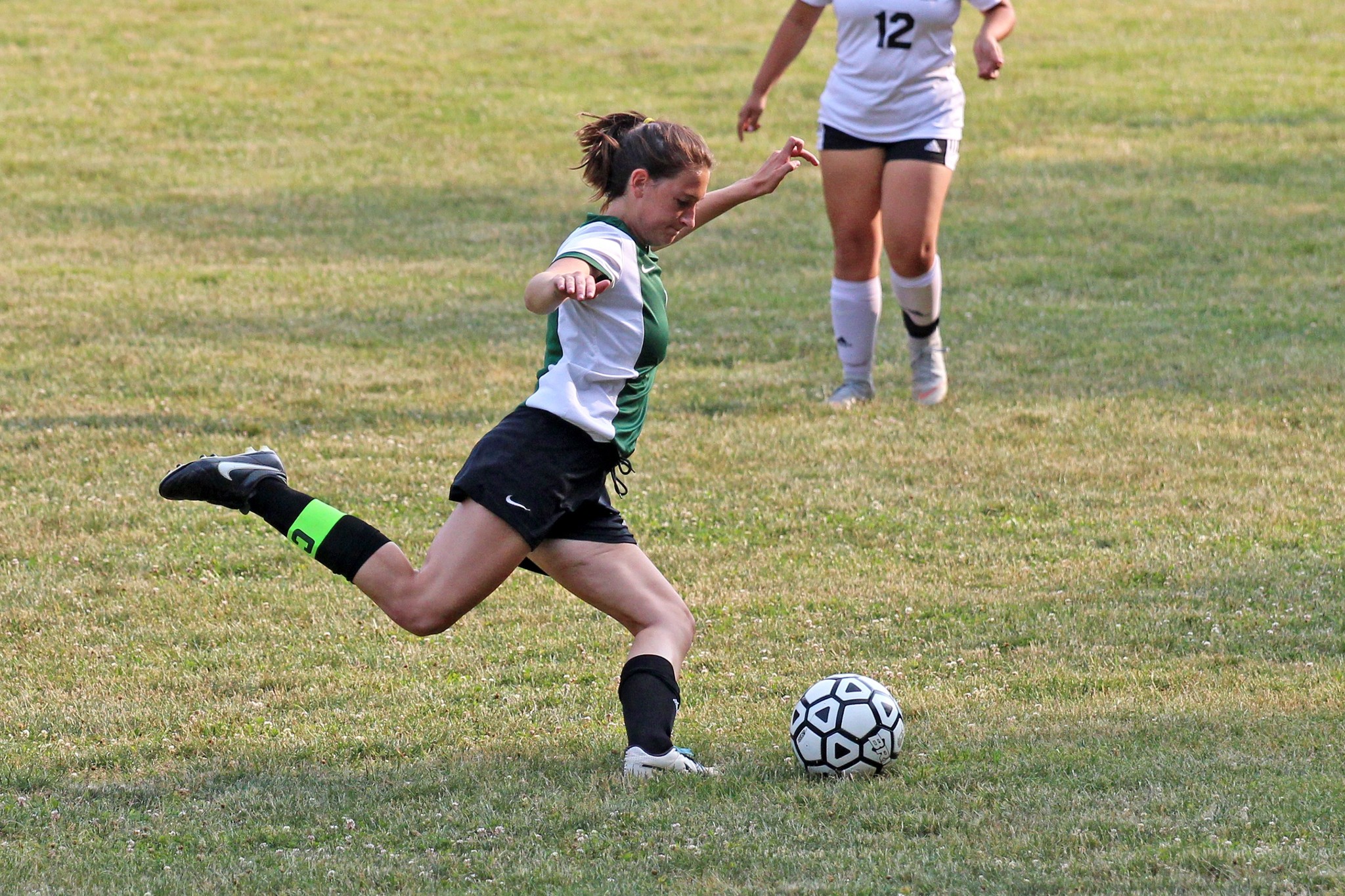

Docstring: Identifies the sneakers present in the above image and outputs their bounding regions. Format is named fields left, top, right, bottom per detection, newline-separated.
left=159, top=446, right=288, bottom=515
left=908, top=329, right=948, bottom=404
left=624, top=743, right=717, bottom=778
left=829, top=379, right=875, bottom=403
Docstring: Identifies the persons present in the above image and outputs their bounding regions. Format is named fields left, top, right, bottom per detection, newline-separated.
left=159, top=110, right=818, bottom=776
left=737, top=0, right=1017, bottom=408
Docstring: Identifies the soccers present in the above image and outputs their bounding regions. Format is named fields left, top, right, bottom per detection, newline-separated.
left=789, top=674, right=904, bottom=780
